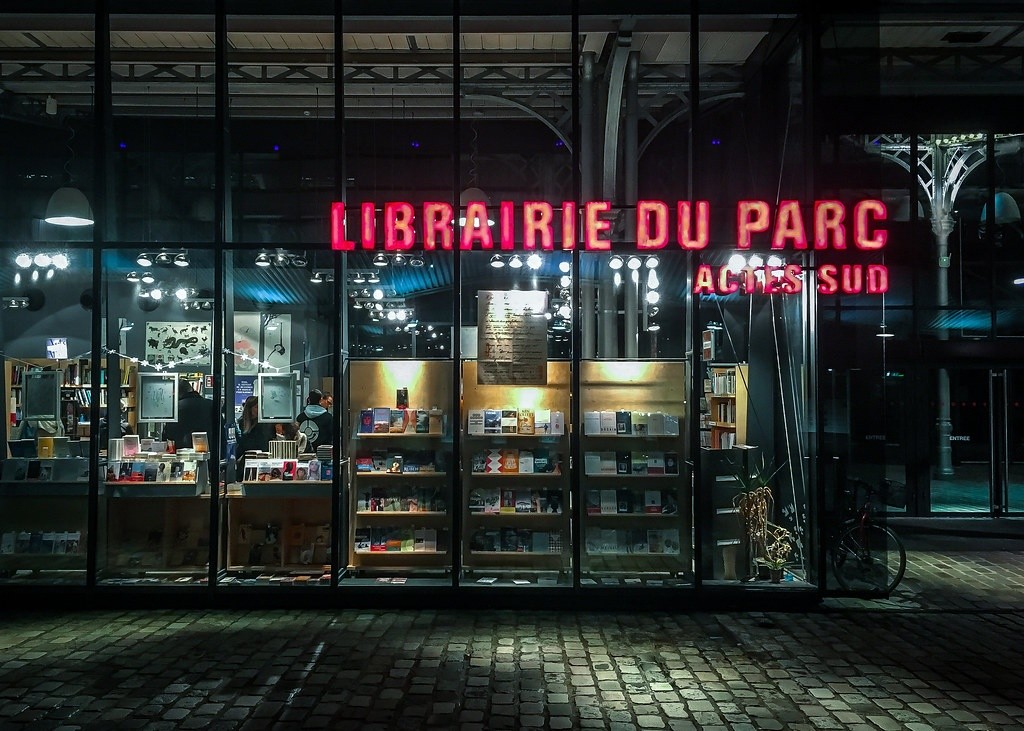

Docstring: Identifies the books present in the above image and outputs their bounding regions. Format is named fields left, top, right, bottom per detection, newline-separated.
left=700, top=370, right=735, bottom=449
left=580, top=578, right=691, bottom=586
left=477, top=577, right=530, bottom=585
left=376, top=578, right=407, bottom=584
left=219, top=524, right=330, bottom=585
left=353, top=386, right=680, bottom=555
left=243, top=440, right=333, bottom=481
left=1, top=366, right=210, bottom=584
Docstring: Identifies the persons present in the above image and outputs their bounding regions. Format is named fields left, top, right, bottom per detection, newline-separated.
left=665, top=457, right=678, bottom=474
left=361, top=414, right=372, bottom=432
left=544, top=423, right=548, bottom=433
left=308, top=461, right=320, bottom=479
left=163, top=379, right=228, bottom=461
left=220, top=389, right=333, bottom=470
left=100, top=397, right=134, bottom=450
left=376, top=497, right=383, bottom=511
left=624, top=530, right=632, bottom=552
left=390, top=498, right=395, bottom=510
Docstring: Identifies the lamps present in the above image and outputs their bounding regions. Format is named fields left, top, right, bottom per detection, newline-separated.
left=128, top=249, right=308, bottom=311
left=43, top=127, right=95, bottom=224
left=450, top=126, right=493, bottom=226
left=979, top=157, right=1021, bottom=224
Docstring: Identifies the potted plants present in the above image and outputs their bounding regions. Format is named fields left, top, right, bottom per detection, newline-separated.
left=729, top=452, right=792, bottom=582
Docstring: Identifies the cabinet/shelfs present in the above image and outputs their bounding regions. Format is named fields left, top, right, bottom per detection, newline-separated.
left=1, top=478, right=335, bottom=607
left=706, top=366, right=749, bottom=448
left=3, top=358, right=138, bottom=441
left=336, top=429, right=697, bottom=611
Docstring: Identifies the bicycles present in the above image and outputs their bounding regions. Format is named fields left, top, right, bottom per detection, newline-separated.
left=822, top=472, right=917, bottom=599
